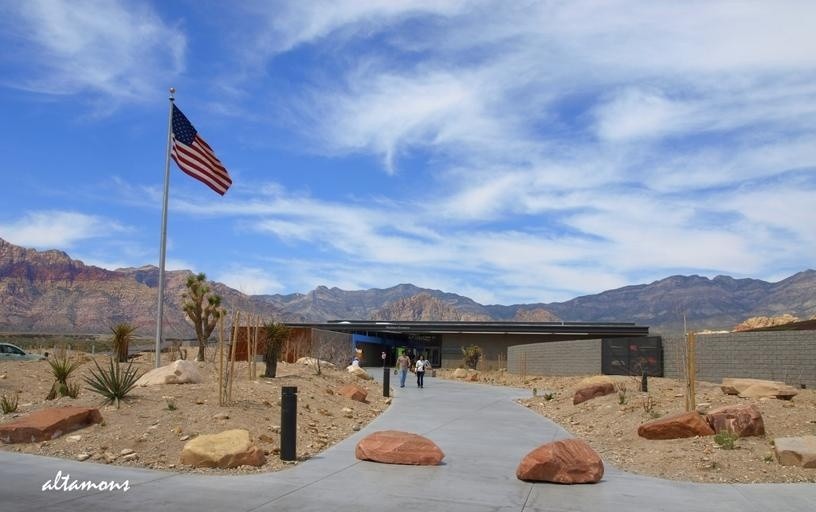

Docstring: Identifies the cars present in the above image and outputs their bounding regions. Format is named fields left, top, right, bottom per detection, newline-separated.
left=0, top=343, right=48, bottom=363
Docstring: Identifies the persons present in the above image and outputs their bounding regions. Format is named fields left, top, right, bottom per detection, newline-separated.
left=352, top=356, right=360, bottom=368
left=404, top=349, right=430, bottom=374
left=396, top=350, right=411, bottom=388
left=414, top=353, right=430, bottom=388
left=381, top=351, right=386, bottom=369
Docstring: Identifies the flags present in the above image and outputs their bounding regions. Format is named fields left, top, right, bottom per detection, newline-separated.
left=167, top=84, right=232, bottom=198
